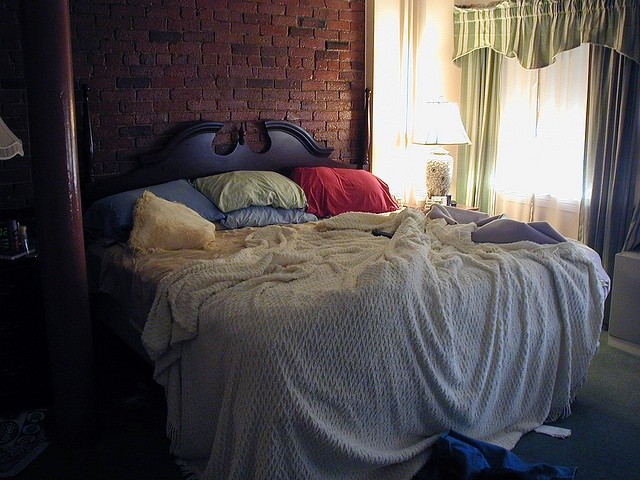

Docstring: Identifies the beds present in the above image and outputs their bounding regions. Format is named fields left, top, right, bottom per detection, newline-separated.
left=84, top=86, right=610, bottom=479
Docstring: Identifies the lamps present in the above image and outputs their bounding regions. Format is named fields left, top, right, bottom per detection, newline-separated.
left=409, top=96, right=472, bottom=197
left=0, top=117, right=25, bottom=161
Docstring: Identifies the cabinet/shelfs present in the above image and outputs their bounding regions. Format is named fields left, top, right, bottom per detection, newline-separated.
left=610, top=249, right=639, bottom=345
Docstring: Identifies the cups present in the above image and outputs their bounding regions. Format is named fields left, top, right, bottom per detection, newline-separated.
left=7, top=219, right=20, bottom=254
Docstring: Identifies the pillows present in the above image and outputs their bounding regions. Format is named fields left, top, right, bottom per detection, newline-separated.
left=93, top=178, right=225, bottom=248
left=289, top=168, right=400, bottom=218
left=128, top=190, right=216, bottom=251
left=221, top=205, right=319, bottom=229
left=195, top=170, right=308, bottom=212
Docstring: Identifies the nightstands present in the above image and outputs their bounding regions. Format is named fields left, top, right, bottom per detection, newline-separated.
left=404, top=202, right=479, bottom=214
left=0, top=229, right=50, bottom=377
